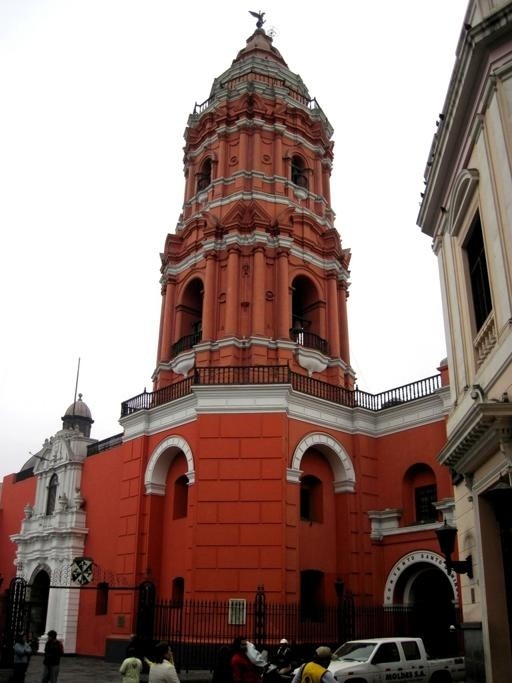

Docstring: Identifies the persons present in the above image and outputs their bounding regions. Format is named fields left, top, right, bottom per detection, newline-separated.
left=41, top=628, right=64, bottom=682
left=142, top=645, right=175, bottom=669
left=211, top=634, right=341, bottom=683
left=148, top=640, right=180, bottom=682
left=11, top=628, right=32, bottom=682
left=118, top=644, right=143, bottom=683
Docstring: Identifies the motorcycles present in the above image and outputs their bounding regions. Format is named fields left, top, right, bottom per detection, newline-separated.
left=260, top=654, right=299, bottom=683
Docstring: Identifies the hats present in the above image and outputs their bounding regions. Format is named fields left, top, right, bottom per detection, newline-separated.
left=316, top=647, right=337, bottom=659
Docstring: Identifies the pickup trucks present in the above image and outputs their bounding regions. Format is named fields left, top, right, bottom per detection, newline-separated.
left=326, top=635, right=469, bottom=683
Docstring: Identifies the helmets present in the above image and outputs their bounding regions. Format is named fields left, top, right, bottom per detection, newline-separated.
left=280, top=639, right=288, bottom=643
